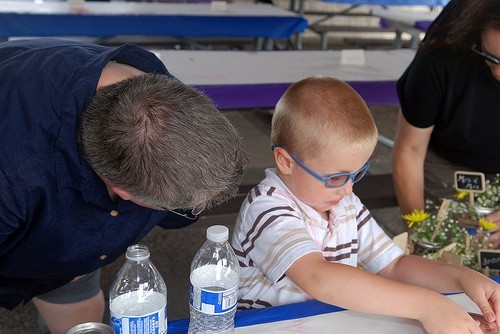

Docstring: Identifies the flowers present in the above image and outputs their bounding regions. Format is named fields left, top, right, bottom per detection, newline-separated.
left=399, top=173, right=500, bottom=269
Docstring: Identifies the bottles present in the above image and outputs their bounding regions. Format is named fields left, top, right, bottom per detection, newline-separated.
left=109, top=245, right=168, bottom=334
left=187, top=225, right=240, bottom=334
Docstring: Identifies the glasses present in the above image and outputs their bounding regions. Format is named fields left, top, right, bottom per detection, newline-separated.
left=271, top=144, right=370, bottom=188
left=470, top=41, right=500, bottom=66
left=156, top=203, right=207, bottom=220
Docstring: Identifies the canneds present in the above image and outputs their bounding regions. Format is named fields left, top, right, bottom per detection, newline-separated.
left=66, top=321, right=116, bottom=334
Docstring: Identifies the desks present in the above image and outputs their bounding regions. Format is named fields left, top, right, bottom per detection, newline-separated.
left=167, top=275, right=500, bottom=334
left=0, top=0, right=452, bottom=109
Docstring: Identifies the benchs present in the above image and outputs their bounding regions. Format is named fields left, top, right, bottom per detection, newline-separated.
left=309, top=24, right=404, bottom=50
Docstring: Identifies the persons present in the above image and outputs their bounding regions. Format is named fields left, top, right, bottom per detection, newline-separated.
left=392, top=0, right=500, bottom=250
left=0, top=38, right=249, bottom=334
left=231, top=75, right=500, bottom=334
